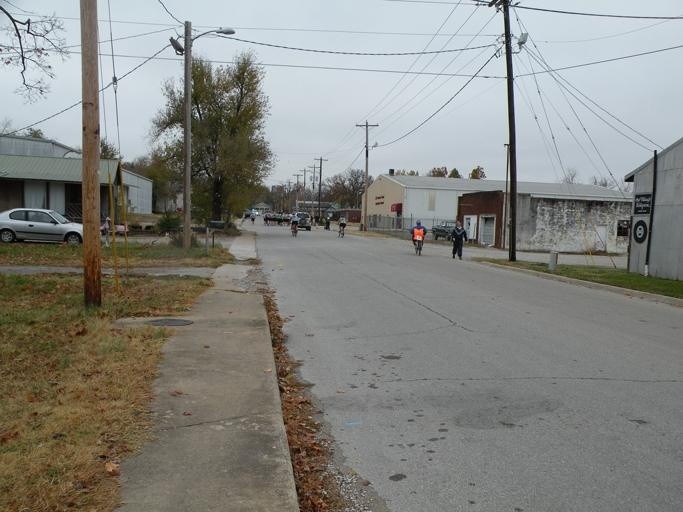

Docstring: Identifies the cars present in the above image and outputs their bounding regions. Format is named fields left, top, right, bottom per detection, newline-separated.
left=432, top=221, right=456, bottom=242
left=0, top=206, right=101, bottom=247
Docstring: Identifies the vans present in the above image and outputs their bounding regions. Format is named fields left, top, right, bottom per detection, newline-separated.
left=295, top=211, right=311, bottom=231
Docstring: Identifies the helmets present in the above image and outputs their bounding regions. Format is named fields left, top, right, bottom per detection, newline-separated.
left=416, top=220, right=420, bottom=224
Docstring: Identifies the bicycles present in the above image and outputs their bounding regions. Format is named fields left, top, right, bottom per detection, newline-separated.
left=337, top=224, right=344, bottom=239
left=291, top=223, right=298, bottom=239
left=414, top=235, right=423, bottom=256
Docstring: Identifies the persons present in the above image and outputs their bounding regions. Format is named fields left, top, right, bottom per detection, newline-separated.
left=410, top=220, right=427, bottom=247
left=263, top=210, right=292, bottom=228
left=288, top=213, right=298, bottom=233
left=451, top=220, right=468, bottom=260
left=336, top=215, right=346, bottom=234
left=250, top=211, right=255, bottom=225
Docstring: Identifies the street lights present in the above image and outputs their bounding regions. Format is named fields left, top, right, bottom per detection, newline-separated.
left=182, top=20, right=234, bottom=250
